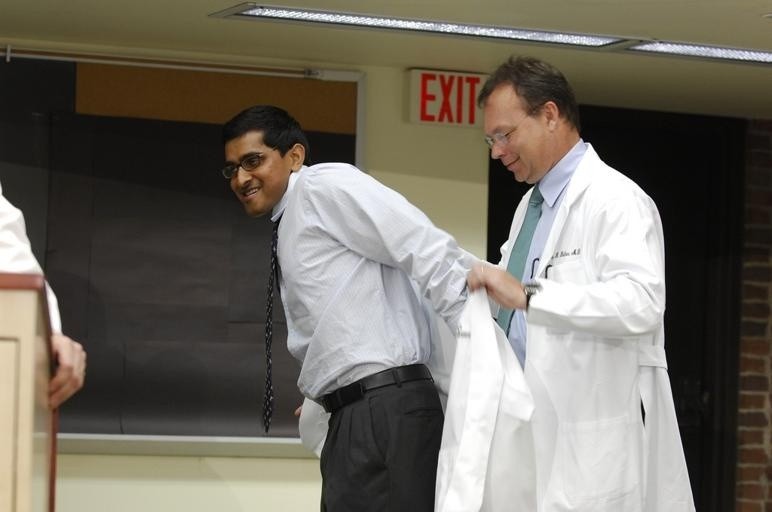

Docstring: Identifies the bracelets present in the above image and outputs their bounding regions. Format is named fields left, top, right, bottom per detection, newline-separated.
left=524, top=282, right=537, bottom=314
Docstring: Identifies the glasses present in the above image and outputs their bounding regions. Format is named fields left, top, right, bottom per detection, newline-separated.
left=219, top=143, right=285, bottom=180
left=484, top=100, right=553, bottom=148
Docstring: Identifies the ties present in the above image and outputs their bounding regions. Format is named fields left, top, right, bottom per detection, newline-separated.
left=496, top=181, right=546, bottom=342
left=261, top=214, right=284, bottom=435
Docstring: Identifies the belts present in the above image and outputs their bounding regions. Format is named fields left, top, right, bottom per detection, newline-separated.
left=312, top=360, right=434, bottom=416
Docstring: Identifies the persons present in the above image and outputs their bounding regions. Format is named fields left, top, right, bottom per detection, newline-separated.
left=465, top=52, right=697, bottom=512
left=219, top=104, right=536, bottom=512
left=0, top=185, right=87, bottom=410
